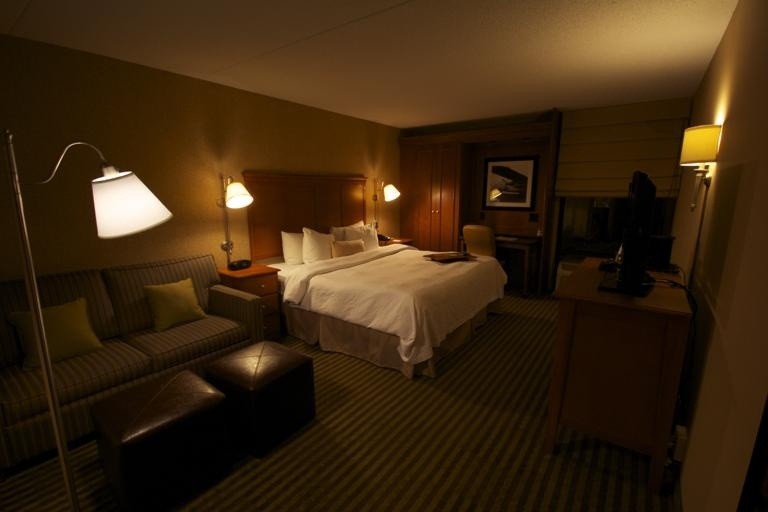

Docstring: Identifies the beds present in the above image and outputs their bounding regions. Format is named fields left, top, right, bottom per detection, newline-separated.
left=240, top=171, right=510, bottom=380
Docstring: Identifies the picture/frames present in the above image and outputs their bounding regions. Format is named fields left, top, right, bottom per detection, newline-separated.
left=483, top=154, right=538, bottom=212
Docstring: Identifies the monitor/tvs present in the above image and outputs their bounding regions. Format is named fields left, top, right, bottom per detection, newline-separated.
left=597, top=170, right=656, bottom=298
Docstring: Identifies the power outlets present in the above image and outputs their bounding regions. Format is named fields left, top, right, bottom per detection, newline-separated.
left=675, top=426, right=688, bottom=464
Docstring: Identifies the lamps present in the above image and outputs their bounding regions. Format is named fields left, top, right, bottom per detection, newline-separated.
left=372, top=176, right=401, bottom=241
left=489, top=186, right=501, bottom=202
left=0, top=128, right=165, bottom=512
left=216, top=176, right=255, bottom=271
left=680, top=123, right=722, bottom=290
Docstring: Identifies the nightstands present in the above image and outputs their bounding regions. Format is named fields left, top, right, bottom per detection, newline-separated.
left=379, top=238, right=415, bottom=248
left=217, top=265, right=283, bottom=343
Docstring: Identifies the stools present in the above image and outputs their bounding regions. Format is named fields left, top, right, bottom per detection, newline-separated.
left=91, top=370, right=227, bottom=512
left=205, top=342, right=315, bottom=458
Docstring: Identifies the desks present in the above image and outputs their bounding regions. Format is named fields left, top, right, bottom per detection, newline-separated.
left=494, top=236, right=542, bottom=297
left=547, top=257, right=693, bottom=492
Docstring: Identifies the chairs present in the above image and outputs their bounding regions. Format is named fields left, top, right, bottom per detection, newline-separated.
left=462, top=224, right=496, bottom=258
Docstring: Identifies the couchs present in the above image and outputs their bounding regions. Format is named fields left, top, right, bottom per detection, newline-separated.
left=1, top=253, right=262, bottom=466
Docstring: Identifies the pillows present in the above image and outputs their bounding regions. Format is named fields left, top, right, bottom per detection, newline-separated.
left=8, top=297, right=106, bottom=372
left=142, top=278, right=208, bottom=333
left=280, top=219, right=379, bottom=266
